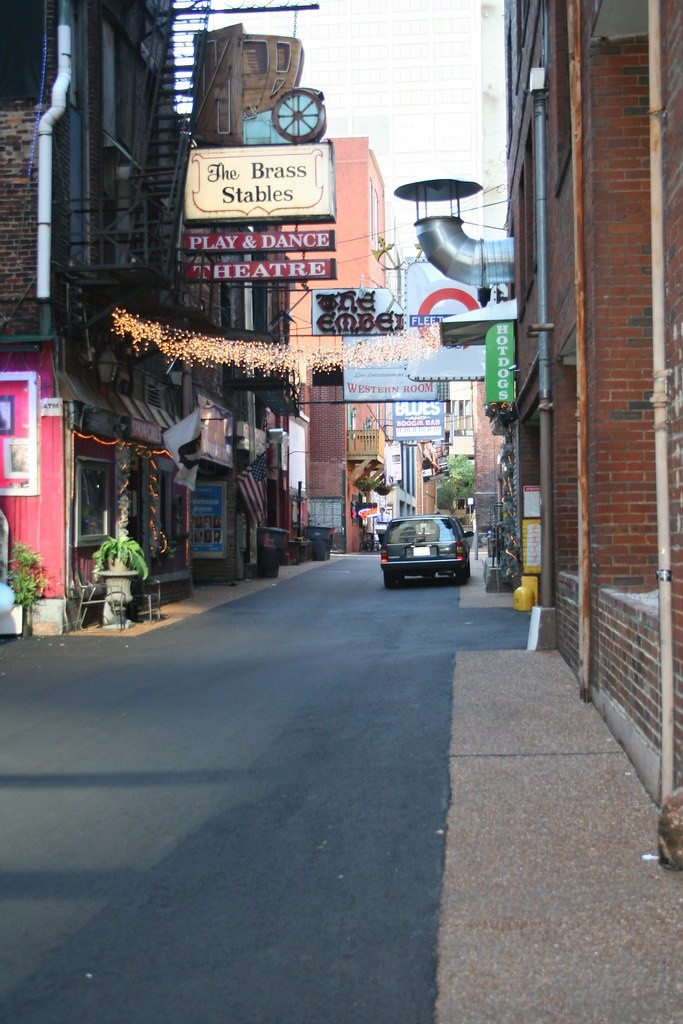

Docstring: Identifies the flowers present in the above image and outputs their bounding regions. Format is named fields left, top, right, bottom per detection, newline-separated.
left=373, top=481, right=394, bottom=491
left=353, top=475, right=376, bottom=491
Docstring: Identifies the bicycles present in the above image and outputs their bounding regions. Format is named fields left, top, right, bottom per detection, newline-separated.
left=359, top=537, right=373, bottom=552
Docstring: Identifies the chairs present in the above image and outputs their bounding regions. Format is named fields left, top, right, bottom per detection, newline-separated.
left=130, top=581, right=163, bottom=624
left=74, top=566, right=127, bottom=632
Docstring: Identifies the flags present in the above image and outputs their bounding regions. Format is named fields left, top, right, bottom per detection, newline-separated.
left=392, top=402, right=445, bottom=441
left=163, top=410, right=202, bottom=491
left=420, top=440, right=450, bottom=483
left=236, top=452, right=269, bottom=523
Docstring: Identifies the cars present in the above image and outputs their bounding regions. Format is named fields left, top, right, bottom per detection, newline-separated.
left=380, top=514, right=475, bottom=587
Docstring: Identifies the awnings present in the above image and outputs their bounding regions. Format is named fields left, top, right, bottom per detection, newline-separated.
left=439, top=298, right=517, bottom=347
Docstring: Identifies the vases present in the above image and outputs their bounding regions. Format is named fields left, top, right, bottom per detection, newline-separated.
left=363, top=486, right=370, bottom=491
left=377, top=490, right=389, bottom=495
